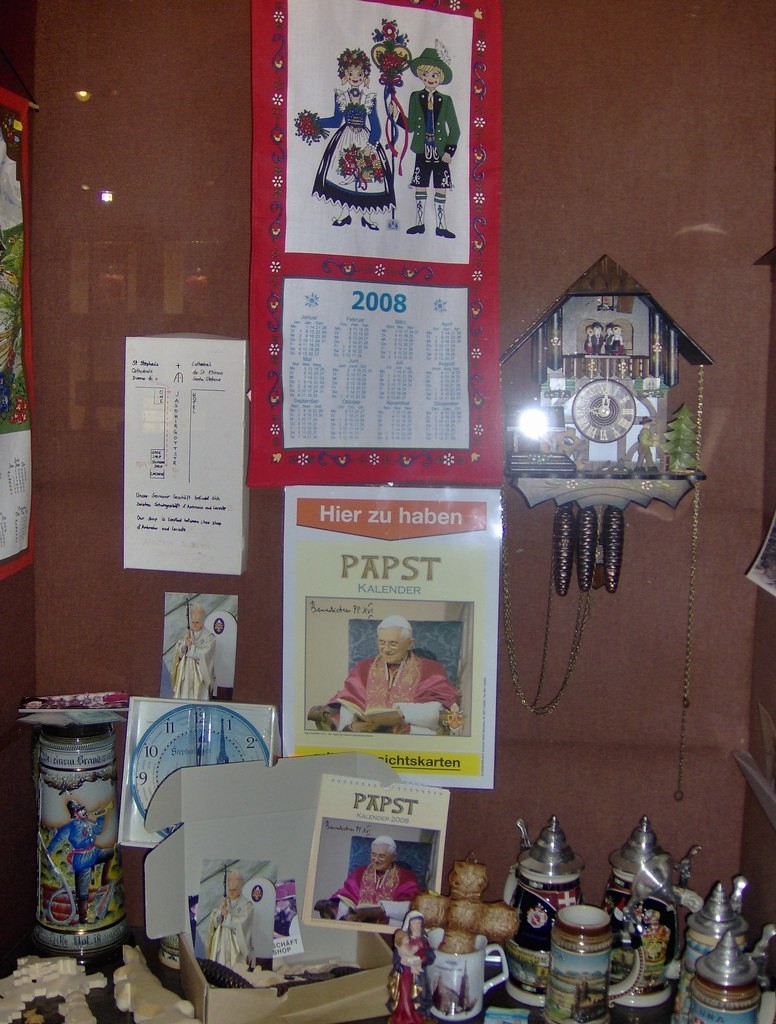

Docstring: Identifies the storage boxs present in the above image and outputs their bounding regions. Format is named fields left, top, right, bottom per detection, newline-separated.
left=139, top=756, right=400, bottom=1023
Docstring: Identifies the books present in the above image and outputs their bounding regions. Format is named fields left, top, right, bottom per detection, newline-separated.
left=336, top=699, right=404, bottom=726
left=337, top=895, right=384, bottom=918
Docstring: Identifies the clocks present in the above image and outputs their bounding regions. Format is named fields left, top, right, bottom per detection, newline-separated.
left=573, top=377, right=638, bottom=445
left=117, top=694, right=277, bottom=850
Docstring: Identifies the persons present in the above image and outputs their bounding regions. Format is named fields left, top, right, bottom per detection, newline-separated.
left=585, top=322, right=623, bottom=355
left=395, top=929, right=424, bottom=973
left=205, top=871, right=255, bottom=969
left=385, top=910, right=436, bottom=1024
left=169, top=604, right=216, bottom=701
left=635, top=416, right=659, bottom=471
left=327, top=614, right=461, bottom=735
left=329, top=835, right=422, bottom=928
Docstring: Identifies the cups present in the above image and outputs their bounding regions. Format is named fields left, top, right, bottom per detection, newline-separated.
left=545, top=904, right=645, bottom=1024
left=426, top=925, right=510, bottom=1021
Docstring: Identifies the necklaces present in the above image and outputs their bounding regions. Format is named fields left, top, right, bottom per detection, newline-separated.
left=229, top=895, right=242, bottom=908
left=192, top=630, right=202, bottom=640
